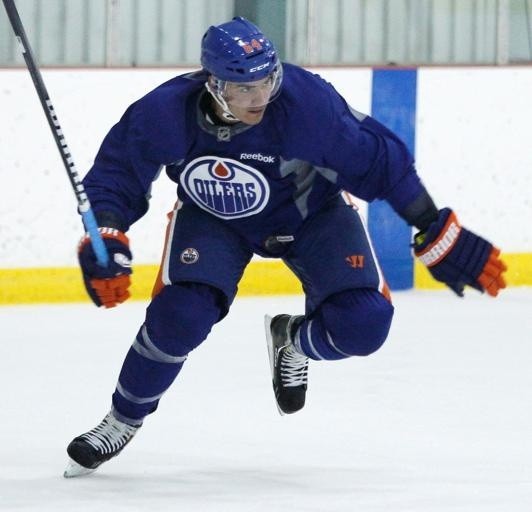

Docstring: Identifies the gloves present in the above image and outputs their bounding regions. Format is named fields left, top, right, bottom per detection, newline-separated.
left=77, top=218, right=132, bottom=309
left=413, top=207, right=508, bottom=298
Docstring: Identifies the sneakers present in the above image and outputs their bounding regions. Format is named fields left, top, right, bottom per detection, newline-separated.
left=270, top=313, right=307, bottom=413
left=67, top=409, right=143, bottom=470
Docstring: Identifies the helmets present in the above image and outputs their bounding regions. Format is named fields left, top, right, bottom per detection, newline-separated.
left=200, top=15, right=284, bottom=108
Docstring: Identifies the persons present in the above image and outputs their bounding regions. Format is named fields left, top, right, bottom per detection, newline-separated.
left=67, top=15, right=508, bottom=471
